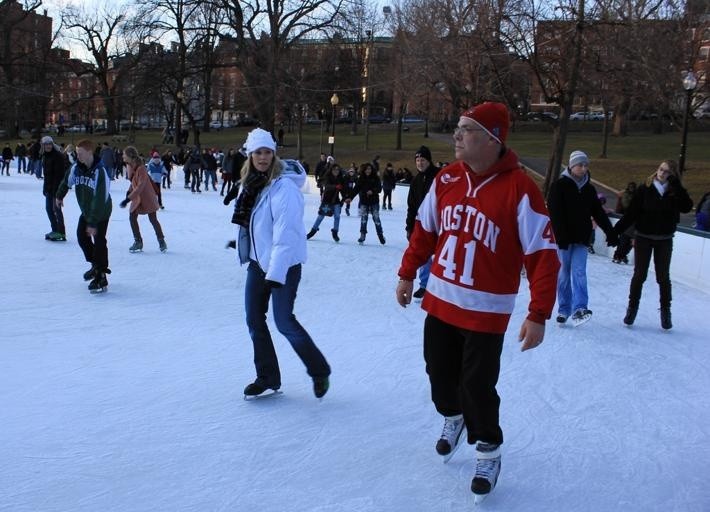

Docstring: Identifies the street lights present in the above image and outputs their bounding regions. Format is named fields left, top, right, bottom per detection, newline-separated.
left=330, top=93, right=339, bottom=157
left=676, top=67, right=696, bottom=176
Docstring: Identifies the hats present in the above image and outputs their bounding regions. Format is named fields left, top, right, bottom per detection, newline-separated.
left=414, top=145, right=434, bottom=161
left=40, top=136, right=62, bottom=155
left=461, top=99, right=509, bottom=143
left=123, top=146, right=141, bottom=156
left=568, top=149, right=589, bottom=172
left=245, top=126, right=277, bottom=154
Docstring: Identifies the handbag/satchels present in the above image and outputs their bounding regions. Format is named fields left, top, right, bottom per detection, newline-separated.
left=150, top=179, right=160, bottom=194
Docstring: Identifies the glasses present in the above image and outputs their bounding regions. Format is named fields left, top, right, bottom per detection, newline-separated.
left=452, top=124, right=485, bottom=135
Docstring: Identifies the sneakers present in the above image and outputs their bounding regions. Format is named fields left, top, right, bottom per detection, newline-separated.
left=556, top=304, right=673, bottom=330
left=470, top=439, right=503, bottom=496
left=160, top=183, right=228, bottom=210
left=312, top=365, right=331, bottom=399
left=1, top=169, right=44, bottom=182
left=45, top=230, right=169, bottom=290
left=411, top=284, right=426, bottom=299
left=244, top=373, right=281, bottom=396
left=436, top=408, right=473, bottom=456
left=305, top=203, right=392, bottom=244
left=589, top=244, right=630, bottom=263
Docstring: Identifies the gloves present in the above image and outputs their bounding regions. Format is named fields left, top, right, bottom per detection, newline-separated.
left=224, top=237, right=237, bottom=252
left=605, top=225, right=619, bottom=247
left=667, top=175, right=679, bottom=188
left=556, top=239, right=567, bottom=250
left=119, top=191, right=131, bottom=209
left=264, top=278, right=281, bottom=291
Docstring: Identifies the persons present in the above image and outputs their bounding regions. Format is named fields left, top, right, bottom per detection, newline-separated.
left=588, top=193, right=616, bottom=254
left=179, top=144, right=249, bottom=196
left=41, top=137, right=77, bottom=242
left=613, top=182, right=638, bottom=264
left=14, top=137, right=43, bottom=179
left=345, top=153, right=396, bottom=244
left=56, top=140, right=111, bottom=294
left=548, top=150, right=619, bottom=323
left=694, top=192, right=710, bottom=233
left=110, top=146, right=181, bottom=254
left=396, top=100, right=560, bottom=494
left=606, top=160, right=693, bottom=329
left=1, top=143, right=14, bottom=176
left=399, top=145, right=448, bottom=297
left=236, top=128, right=331, bottom=397
left=297, top=154, right=360, bottom=242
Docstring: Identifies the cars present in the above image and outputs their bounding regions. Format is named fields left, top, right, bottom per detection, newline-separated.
left=514, top=107, right=710, bottom=122
left=0, top=122, right=145, bottom=139
left=193, top=115, right=427, bottom=129
